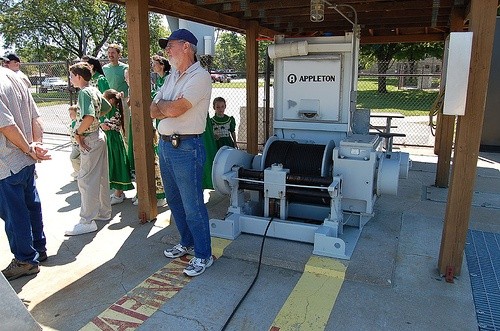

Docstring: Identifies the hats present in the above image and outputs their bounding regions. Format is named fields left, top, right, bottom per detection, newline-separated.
left=159, top=29, right=198, bottom=48
left=0, top=54, right=20, bottom=62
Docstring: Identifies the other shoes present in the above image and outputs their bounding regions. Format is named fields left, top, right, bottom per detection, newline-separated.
left=110, top=192, right=126, bottom=204
left=131, top=196, right=138, bottom=206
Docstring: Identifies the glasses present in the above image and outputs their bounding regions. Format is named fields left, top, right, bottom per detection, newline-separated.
left=5, top=62, right=15, bottom=64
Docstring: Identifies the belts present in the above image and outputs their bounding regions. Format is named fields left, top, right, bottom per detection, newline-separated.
left=160, top=133, right=198, bottom=142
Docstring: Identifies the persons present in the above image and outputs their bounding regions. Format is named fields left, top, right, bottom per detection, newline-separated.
left=64, top=44, right=138, bottom=235
left=150, top=29, right=239, bottom=276
left=0, top=53, right=52, bottom=282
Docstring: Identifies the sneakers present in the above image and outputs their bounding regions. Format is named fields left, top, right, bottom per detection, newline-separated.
left=184, top=255, right=213, bottom=277
left=65, top=221, right=98, bottom=236
left=93, top=215, right=110, bottom=221
left=38, top=251, right=48, bottom=262
left=1, top=258, right=40, bottom=280
left=164, top=244, right=196, bottom=258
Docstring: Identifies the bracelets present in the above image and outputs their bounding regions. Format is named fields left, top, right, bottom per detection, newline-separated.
left=25, top=146, right=32, bottom=155
left=33, top=142, right=43, bottom=145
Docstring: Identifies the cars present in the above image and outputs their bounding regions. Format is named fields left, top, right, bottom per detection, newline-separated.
left=210, top=74, right=225, bottom=83
left=42, top=78, right=68, bottom=91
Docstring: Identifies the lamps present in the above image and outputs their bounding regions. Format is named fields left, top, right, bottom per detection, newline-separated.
left=309, top=0, right=324, bottom=22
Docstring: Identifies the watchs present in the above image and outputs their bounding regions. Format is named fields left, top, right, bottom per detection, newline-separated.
left=156, top=98, right=161, bottom=104
